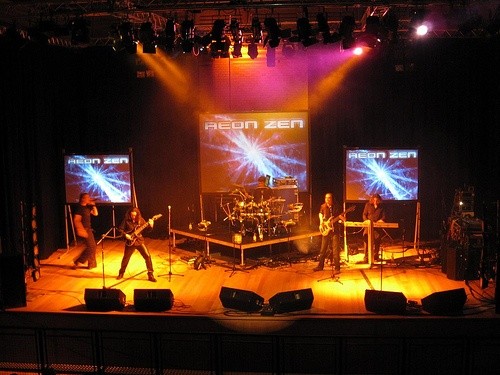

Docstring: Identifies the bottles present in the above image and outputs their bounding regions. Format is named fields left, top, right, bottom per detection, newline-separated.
left=253, top=233, right=257, bottom=242
left=259, top=231, right=264, bottom=242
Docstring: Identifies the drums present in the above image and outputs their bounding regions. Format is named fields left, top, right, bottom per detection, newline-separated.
left=232, top=198, right=285, bottom=235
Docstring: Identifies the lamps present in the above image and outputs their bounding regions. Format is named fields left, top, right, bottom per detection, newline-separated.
left=0, top=6, right=500, bottom=58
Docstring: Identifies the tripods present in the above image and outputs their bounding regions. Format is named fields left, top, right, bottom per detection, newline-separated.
left=158, top=209, right=184, bottom=282
left=317, top=208, right=344, bottom=285
left=397, top=227, right=414, bottom=266
left=224, top=210, right=249, bottom=278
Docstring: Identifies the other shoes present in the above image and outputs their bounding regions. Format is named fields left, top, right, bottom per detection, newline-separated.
left=149, top=276, right=157, bottom=282
left=117, top=273, right=123, bottom=280
left=335, top=270, right=341, bottom=274
left=314, top=267, right=324, bottom=272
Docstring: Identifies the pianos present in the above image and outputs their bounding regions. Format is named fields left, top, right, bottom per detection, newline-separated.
left=344, top=221, right=400, bottom=266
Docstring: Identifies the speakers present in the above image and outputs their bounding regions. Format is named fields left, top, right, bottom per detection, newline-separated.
left=445, top=241, right=484, bottom=281
left=134, top=289, right=174, bottom=311
left=269, top=288, right=314, bottom=313
left=421, top=288, right=467, bottom=315
left=364, top=289, right=407, bottom=314
left=84, top=288, right=126, bottom=309
left=219, top=286, right=264, bottom=311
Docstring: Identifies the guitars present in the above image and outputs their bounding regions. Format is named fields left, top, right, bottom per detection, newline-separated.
left=318, top=206, right=356, bottom=236
left=122, top=213, right=162, bottom=246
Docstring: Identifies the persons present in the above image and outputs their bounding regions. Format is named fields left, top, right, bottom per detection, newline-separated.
left=313, top=193, right=342, bottom=274
left=116, top=208, right=157, bottom=282
left=73, top=193, right=98, bottom=269
left=253, top=176, right=273, bottom=203
left=362, top=194, right=385, bottom=263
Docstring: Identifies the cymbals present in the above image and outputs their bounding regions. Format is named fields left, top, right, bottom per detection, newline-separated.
left=227, top=194, right=240, bottom=196
left=256, top=187, right=269, bottom=190
left=229, top=183, right=244, bottom=188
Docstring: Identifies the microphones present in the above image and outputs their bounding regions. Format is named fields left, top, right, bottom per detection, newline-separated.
left=328, top=204, right=333, bottom=208
left=168, top=206, right=171, bottom=209
